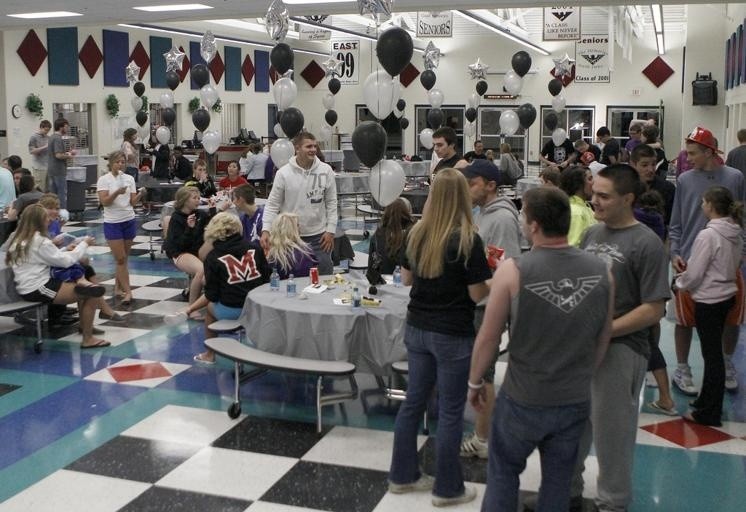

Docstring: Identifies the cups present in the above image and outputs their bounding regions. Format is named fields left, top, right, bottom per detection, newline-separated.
left=269, top=268, right=280, bottom=291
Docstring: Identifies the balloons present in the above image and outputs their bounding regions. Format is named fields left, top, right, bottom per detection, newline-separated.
left=125, top=0, right=575, bottom=207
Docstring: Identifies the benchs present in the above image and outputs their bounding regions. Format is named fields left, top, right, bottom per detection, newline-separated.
left=348, top=250, right=370, bottom=274
left=384, top=361, right=431, bottom=435
left=204, top=318, right=356, bottom=434
left=338, top=192, right=422, bottom=221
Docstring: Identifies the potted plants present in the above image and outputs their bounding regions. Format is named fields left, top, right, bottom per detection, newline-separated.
left=25, top=93, right=44, bottom=120
left=107, top=94, right=120, bottom=119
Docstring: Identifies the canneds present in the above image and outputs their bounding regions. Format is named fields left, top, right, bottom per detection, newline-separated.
left=309, top=267, right=319, bottom=285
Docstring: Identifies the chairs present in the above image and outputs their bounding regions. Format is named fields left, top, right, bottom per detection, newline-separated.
left=1, top=210, right=48, bottom=362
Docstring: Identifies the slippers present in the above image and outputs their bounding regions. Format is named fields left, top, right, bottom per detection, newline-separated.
left=684, top=411, right=697, bottom=421
left=190, top=313, right=206, bottom=322
left=80, top=340, right=110, bottom=348
left=122, top=294, right=132, bottom=305
left=163, top=312, right=187, bottom=325
left=74, top=283, right=105, bottom=297
left=647, top=402, right=678, bottom=415
left=194, top=353, right=216, bottom=364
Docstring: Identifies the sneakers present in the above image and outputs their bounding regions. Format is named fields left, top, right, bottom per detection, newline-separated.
left=99, top=310, right=126, bottom=323
left=433, top=486, right=477, bottom=507
left=672, top=366, right=698, bottom=395
left=460, top=435, right=488, bottom=459
left=724, top=359, right=738, bottom=389
left=390, top=473, right=435, bottom=494
left=79, top=327, right=104, bottom=334
left=525, top=493, right=582, bottom=512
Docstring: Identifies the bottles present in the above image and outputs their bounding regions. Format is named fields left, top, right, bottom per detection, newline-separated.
left=351, top=287, right=361, bottom=310
left=335, top=126, right=339, bottom=133
left=286, top=273, right=297, bottom=298
left=392, top=264, right=401, bottom=285
left=393, top=155, right=396, bottom=161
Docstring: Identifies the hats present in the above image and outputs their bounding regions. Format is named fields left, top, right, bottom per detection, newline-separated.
left=455, top=159, right=498, bottom=181
left=685, top=127, right=725, bottom=153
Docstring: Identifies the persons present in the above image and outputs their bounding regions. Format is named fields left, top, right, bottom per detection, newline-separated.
left=0, top=118, right=746, bottom=512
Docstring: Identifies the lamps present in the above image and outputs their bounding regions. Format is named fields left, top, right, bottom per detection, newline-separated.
left=451, top=10, right=550, bottom=55
left=649, top=3, right=666, bottom=55
left=288, top=14, right=428, bottom=53
left=118, top=21, right=331, bottom=56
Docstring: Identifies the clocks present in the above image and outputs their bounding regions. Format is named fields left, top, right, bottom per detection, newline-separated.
left=12, top=104, right=22, bottom=118
